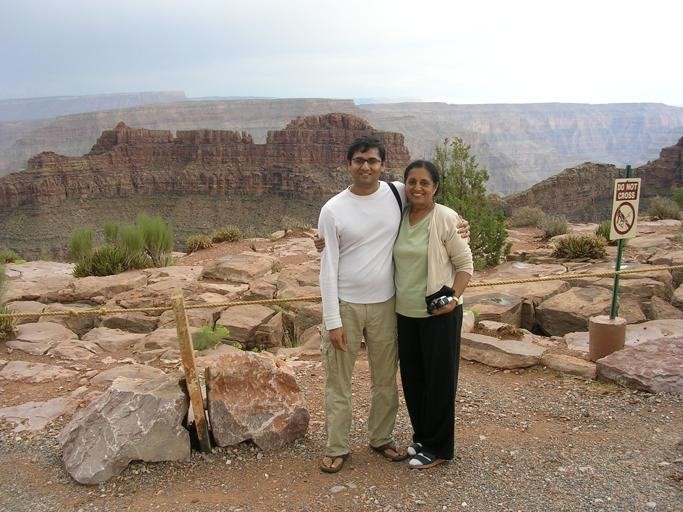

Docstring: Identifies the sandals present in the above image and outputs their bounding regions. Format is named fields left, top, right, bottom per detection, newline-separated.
left=408, top=442, right=446, bottom=469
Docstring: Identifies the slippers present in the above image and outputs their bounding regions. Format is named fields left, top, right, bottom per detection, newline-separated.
left=369, top=442, right=408, bottom=461
left=320, top=456, right=345, bottom=473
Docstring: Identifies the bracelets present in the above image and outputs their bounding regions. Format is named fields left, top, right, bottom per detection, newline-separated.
left=452, top=296, right=459, bottom=305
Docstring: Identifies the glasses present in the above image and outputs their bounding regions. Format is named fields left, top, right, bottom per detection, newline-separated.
left=350, top=157, right=381, bottom=165
